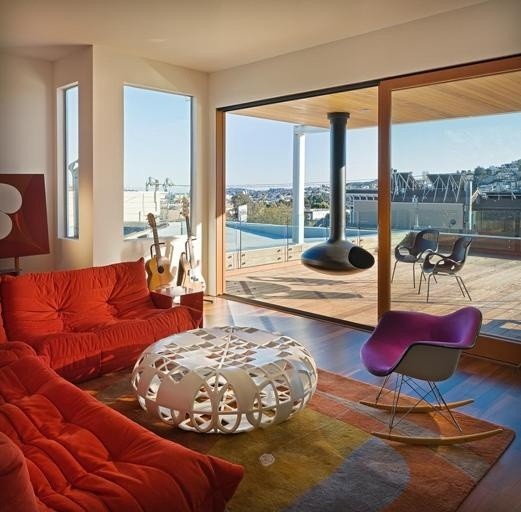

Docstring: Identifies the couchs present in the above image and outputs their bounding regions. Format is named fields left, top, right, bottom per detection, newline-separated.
left=0, top=257, right=200, bottom=382
left=0, top=341, right=244, bottom=511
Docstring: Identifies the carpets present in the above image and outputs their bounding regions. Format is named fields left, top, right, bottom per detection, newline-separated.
left=74, top=344, right=517, bottom=512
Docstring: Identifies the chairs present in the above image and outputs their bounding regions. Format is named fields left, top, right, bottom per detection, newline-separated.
left=391, top=229, right=440, bottom=289
left=360, top=306, right=503, bottom=444
left=418, top=236, right=473, bottom=302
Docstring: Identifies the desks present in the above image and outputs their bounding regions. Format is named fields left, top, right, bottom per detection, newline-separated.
left=152, top=284, right=203, bottom=328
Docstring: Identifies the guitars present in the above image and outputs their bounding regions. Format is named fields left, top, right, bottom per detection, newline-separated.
left=145, top=213, right=174, bottom=290
left=177, top=197, right=206, bottom=291
